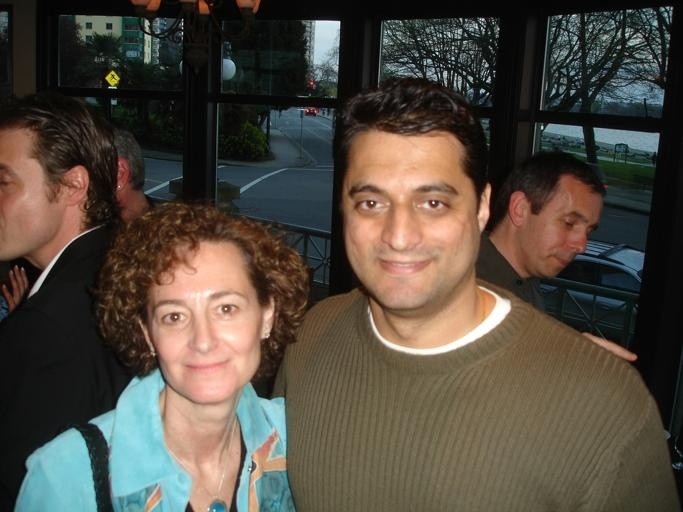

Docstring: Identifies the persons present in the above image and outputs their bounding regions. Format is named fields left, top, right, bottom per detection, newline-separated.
left=264, top=83, right=682, bottom=512
left=0, top=266, right=31, bottom=323
left=13, top=198, right=312, bottom=511
left=0, top=86, right=154, bottom=512
left=474, top=147, right=637, bottom=363
left=104, top=126, right=162, bottom=226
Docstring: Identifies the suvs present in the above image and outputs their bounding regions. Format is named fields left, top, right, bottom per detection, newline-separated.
left=536, top=237, right=645, bottom=347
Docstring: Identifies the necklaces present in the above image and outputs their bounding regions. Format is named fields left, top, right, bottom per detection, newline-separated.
left=171, top=422, right=235, bottom=512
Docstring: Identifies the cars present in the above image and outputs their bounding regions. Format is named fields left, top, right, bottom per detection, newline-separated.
left=304, top=107, right=316, bottom=116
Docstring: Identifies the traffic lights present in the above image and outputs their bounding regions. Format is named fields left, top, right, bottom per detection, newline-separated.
left=309, top=81, right=313, bottom=89
left=307, top=80, right=310, bottom=89
